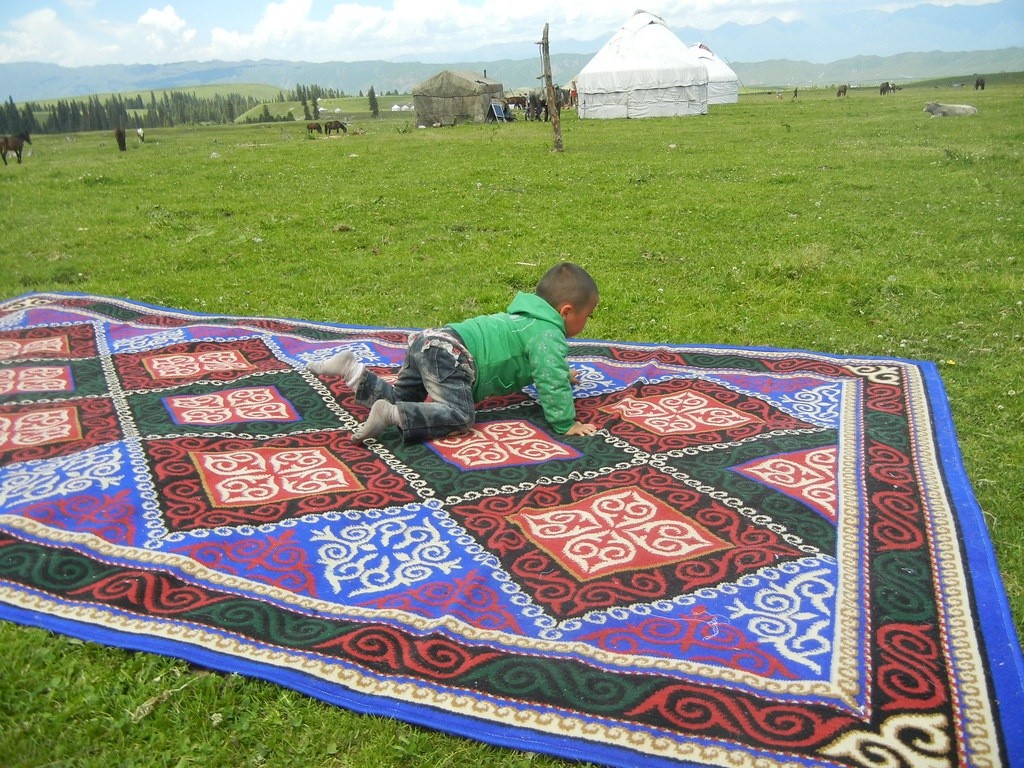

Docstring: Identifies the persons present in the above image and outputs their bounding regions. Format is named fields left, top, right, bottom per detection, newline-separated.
left=793, top=87, right=798, bottom=98
left=523, top=85, right=564, bottom=121
left=305, top=262, right=599, bottom=445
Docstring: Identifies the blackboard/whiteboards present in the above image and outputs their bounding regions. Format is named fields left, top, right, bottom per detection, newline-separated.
left=492, top=103, right=504, bottom=117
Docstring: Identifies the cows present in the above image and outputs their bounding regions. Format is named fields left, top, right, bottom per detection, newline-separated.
left=922, top=100, right=977, bottom=119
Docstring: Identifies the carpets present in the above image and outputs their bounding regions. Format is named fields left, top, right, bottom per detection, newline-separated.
left=0, top=292, right=1024, bottom=768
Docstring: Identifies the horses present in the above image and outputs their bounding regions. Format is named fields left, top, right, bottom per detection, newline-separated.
left=880, top=81, right=903, bottom=96
left=975, top=79, right=985, bottom=91
left=115, top=125, right=145, bottom=151
left=324, top=120, right=347, bottom=135
left=836, top=85, right=847, bottom=97
left=307, top=122, right=323, bottom=134
left=530, top=87, right=571, bottom=122
left=0, top=130, right=32, bottom=166
